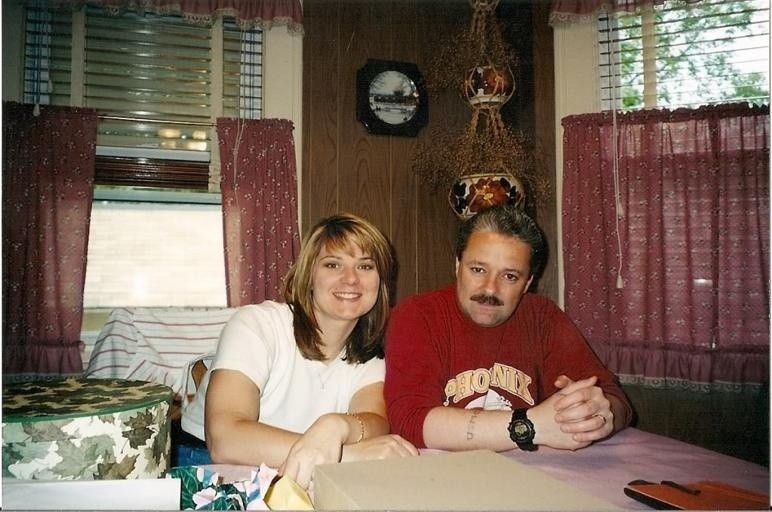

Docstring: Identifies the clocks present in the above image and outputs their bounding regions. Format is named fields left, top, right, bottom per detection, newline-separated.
left=357, top=60, right=427, bottom=133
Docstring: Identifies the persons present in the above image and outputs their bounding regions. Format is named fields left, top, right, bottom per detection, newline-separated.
left=175, top=212, right=422, bottom=492
left=383, top=201, right=634, bottom=454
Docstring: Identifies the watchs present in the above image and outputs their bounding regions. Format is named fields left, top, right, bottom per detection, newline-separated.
left=506, top=407, right=539, bottom=453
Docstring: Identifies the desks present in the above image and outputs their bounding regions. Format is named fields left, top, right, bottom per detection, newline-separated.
left=307, top=419, right=770, bottom=512
left=1, top=374, right=174, bottom=479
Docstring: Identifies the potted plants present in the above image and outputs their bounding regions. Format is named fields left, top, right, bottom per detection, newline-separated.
left=410, top=0, right=554, bottom=224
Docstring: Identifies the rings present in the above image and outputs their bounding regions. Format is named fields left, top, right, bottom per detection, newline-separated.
left=594, top=414, right=606, bottom=426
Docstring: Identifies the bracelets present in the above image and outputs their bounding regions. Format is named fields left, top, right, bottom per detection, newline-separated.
left=344, top=410, right=366, bottom=446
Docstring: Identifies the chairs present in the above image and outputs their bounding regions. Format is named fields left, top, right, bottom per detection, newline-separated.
left=158, top=464, right=314, bottom=510
left=91, top=303, right=229, bottom=409
